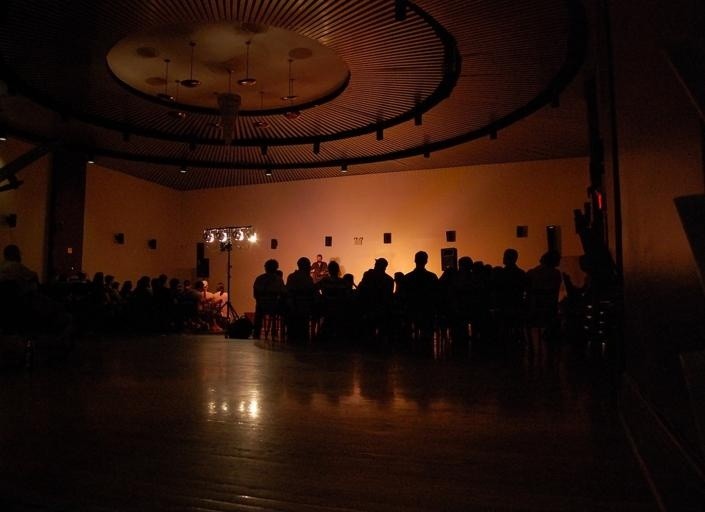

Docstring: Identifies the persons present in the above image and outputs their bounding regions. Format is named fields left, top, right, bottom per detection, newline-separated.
left=1, top=244, right=235, bottom=341
left=252, top=254, right=566, bottom=367
left=557, top=250, right=627, bottom=449
